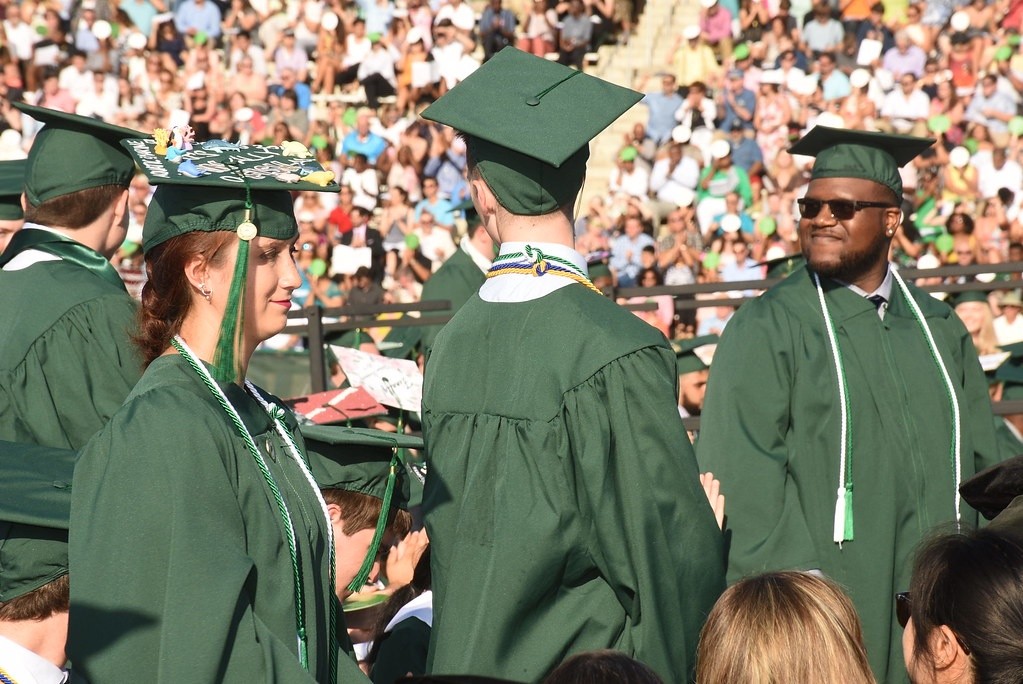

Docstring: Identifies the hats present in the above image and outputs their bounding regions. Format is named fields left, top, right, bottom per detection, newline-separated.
left=0, top=159, right=28, bottom=220
left=297, top=425, right=423, bottom=593
left=445, top=196, right=480, bottom=226
left=419, top=44, right=646, bottom=216
left=786, top=125, right=938, bottom=202
left=0, top=439, right=77, bottom=603
left=119, top=124, right=342, bottom=383
left=668, top=333, right=720, bottom=374
left=10, top=100, right=154, bottom=207
left=944, top=288, right=995, bottom=310
left=585, top=255, right=615, bottom=283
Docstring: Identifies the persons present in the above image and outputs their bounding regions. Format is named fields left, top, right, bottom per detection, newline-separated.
left=693, top=125, right=1023, bottom=684
left=0, top=0, right=1023, bottom=684
left=418, top=44, right=727, bottom=684
left=0, top=100, right=169, bottom=450
left=62, top=123, right=377, bottom=684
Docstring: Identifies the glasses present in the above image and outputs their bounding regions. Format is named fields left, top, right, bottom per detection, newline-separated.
left=796, top=197, right=891, bottom=221
left=895, top=591, right=971, bottom=655
